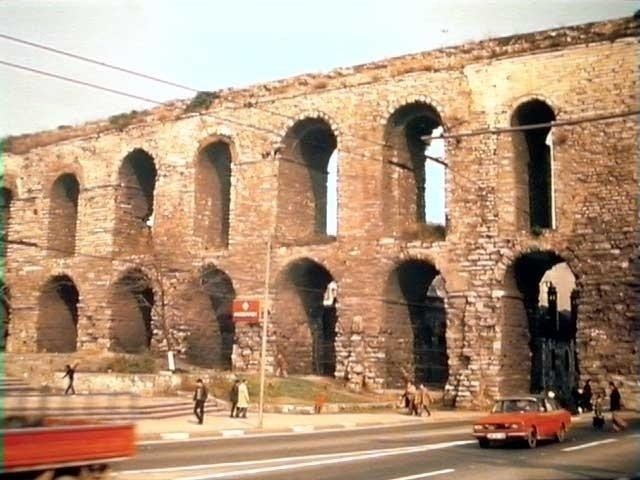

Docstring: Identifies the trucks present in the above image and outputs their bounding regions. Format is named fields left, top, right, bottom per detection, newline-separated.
left=0, top=413, right=137, bottom=480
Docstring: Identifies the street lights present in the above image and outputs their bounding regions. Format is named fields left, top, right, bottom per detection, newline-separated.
left=259, top=138, right=286, bottom=427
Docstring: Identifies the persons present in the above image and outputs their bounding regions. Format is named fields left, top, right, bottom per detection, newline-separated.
left=583, top=379, right=593, bottom=410
left=235, top=378, right=249, bottom=418
left=63, top=361, right=78, bottom=394
left=401, top=381, right=419, bottom=415
left=230, top=379, right=240, bottom=417
left=608, top=381, right=621, bottom=413
left=595, top=402, right=603, bottom=431
left=416, top=383, right=433, bottom=417
left=193, top=378, right=207, bottom=424
left=505, top=401, right=520, bottom=411
left=529, top=402, right=544, bottom=412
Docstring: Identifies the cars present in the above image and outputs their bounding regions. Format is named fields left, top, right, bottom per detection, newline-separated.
left=472, top=394, right=571, bottom=448
